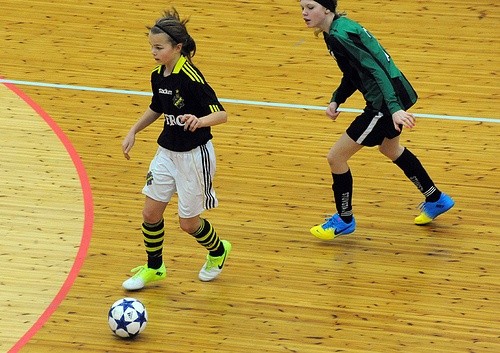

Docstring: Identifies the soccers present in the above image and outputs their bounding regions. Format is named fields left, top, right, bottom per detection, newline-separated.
left=108, top=297, right=148, bottom=337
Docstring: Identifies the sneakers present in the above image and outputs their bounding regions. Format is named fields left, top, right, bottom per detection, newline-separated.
left=309, top=212, right=356, bottom=242
left=122, top=260, right=167, bottom=290
left=199, top=240, right=231, bottom=281
left=413, top=192, right=455, bottom=225
